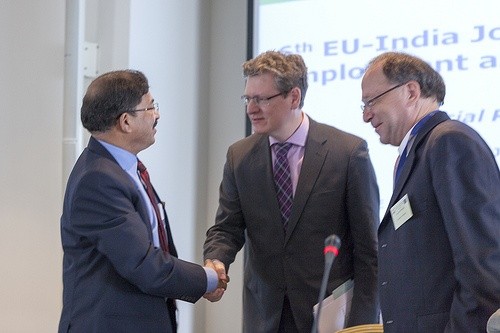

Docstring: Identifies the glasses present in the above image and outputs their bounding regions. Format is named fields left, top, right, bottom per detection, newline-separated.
left=241, top=91, right=286, bottom=106
left=361, top=79, right=407, bottom=111
left=116, top=103, right=160, bottom=123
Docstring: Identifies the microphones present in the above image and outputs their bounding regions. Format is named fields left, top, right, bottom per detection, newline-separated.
left=311, top=234, right=342, bottom=333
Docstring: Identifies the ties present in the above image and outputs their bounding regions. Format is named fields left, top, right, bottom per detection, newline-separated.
left=273, top=142, right=294, bottom=233
left=137, top=160, right=169, bottom=253
left=393, top=145, right=409, bottom=188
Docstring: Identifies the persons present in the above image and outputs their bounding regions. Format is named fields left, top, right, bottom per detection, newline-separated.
left=360, top=52, right=500, bottom=333
left=202, top=51, right=380, bottom=333
left=57, top=70, right=231, bottom=333
